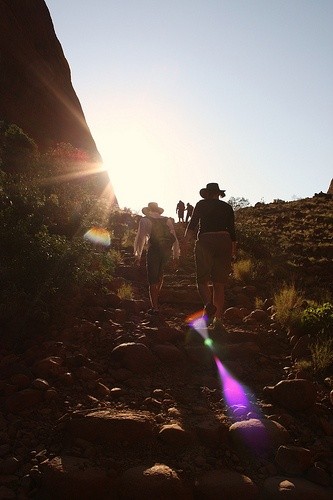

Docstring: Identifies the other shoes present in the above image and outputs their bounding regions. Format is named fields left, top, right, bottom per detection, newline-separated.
left=202, top=304, right=217, bottom=326
left=147, top=307, right=159, bottom=314
left=212, top=318, right=223, bottom=329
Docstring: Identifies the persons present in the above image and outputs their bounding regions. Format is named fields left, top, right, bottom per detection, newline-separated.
left=176, top=201, right=185, bottom=223
left=132, top=203, right=182, bottom=311
left=179, top=183, right=237, bottom=328
left=185, top=203, right=194, bottom=222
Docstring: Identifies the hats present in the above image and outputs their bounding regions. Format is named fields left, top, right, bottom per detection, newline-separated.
left=142, top=203, right=164, bottom=215
left=199, top=182, right=226, bottom=198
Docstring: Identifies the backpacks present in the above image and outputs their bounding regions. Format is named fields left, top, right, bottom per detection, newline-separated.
left=146, top=216, right=176, bottom=251
